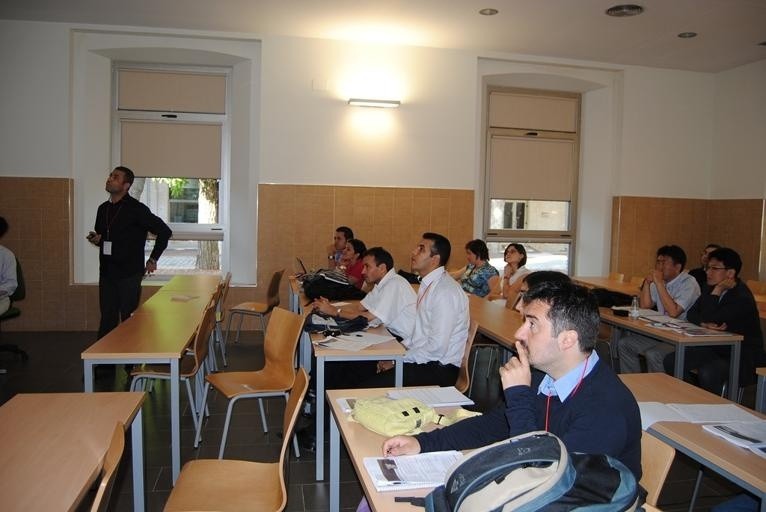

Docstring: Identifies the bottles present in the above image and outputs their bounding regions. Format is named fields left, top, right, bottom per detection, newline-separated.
left=629, top=297, right=641, bottom=322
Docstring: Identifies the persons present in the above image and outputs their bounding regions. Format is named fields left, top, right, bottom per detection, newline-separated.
left=688, top=244, right=722, bottom=296
left=383, top=281, right=642, bottom=482
left=296, top=226, right=532, bottom=452
left=0, top=216, right=18, bottom=316
left=515, top=271, right=573, bottom=320
left=81, top=167, right=172, bottom=382
left=663, top=248, right=766, bottom=397
left=618, top=245, right=701, bottom=374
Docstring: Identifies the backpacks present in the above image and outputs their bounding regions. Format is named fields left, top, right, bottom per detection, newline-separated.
left=402, top=431, right=646, bottom=512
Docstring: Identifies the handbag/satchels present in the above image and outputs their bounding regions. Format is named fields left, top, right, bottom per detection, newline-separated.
left=348, top=397, right=439, bottom=437
left=304, top=312, right=369, bottom=333
left=304, top=274, right=367, bottom=301
